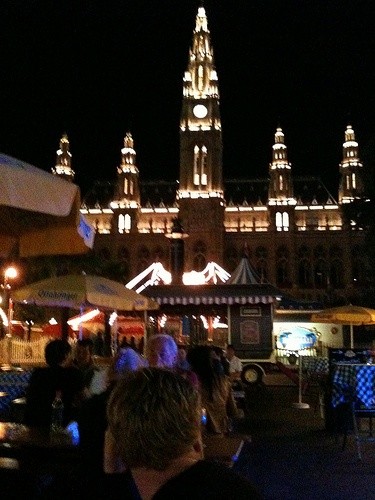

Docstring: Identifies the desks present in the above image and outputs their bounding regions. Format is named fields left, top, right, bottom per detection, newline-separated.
left=332, top=362, right=375, bottom=460
left=1, top=376, right=245, bottom=470
left=303, top=356, right=330, bottom=418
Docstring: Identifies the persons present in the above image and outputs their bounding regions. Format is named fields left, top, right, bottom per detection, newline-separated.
left=27, top=331, right=245, bottom=454
left=106, top=365, right=259, bottom=500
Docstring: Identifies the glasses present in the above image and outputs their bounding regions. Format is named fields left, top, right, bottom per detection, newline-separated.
left=150, top=352, right=172, bottom=359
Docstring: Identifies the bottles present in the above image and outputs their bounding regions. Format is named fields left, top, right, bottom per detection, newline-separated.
left=50, top=391, right=63, bottom=430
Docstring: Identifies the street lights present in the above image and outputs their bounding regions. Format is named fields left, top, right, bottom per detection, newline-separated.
left=4, top=267, right=19, bottom=318
